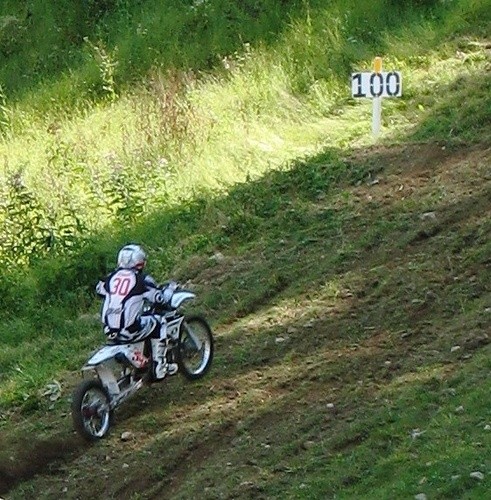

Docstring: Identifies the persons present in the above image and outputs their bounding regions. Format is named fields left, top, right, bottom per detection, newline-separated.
left=100, top=244, right=181, bottom=377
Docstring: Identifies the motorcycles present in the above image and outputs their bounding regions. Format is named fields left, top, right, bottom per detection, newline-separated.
left=72, top=277, right=213, bottom=440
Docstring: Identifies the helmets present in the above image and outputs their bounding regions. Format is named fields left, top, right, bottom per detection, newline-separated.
left=116, top=244, right=146, bottom=268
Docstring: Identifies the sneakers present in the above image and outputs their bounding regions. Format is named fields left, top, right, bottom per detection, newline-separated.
left=155, top=363, right=179, bottom=379
